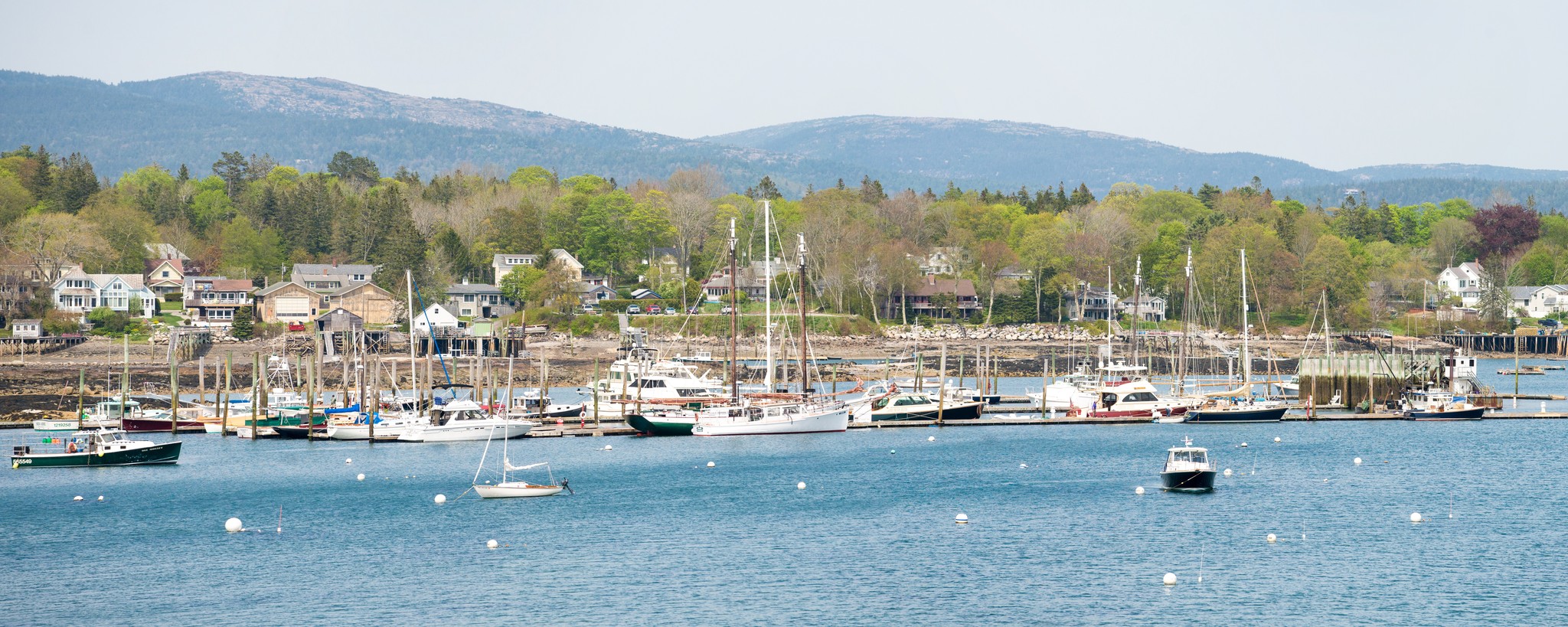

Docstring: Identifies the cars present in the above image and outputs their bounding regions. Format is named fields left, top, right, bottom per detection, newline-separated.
left=664, top=307, right=676, bottom=315
left=686, top=306, right=699, bottom=315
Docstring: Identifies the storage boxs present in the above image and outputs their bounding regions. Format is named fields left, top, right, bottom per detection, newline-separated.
left=289, top=319, right=300, bottom=325
left=1380, top=399, right=1406, bottom=410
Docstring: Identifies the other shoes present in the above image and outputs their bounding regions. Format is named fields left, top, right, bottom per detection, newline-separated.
left=1166, top=416, right=1171, bottom=417
left=1093, top=417, right=1096, bottom=418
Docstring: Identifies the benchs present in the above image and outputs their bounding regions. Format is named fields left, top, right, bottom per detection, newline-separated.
left=751, top=416, right=761, bottom=421
left=166, top=326, right=175, bottom=330
left=1018, top=320, right=1024, bottom=323
left=1070, top=409, right=1081, bottom=415
left=962, top=320, right=969, bottom=321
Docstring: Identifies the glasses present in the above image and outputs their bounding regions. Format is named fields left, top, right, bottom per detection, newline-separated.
left=1249, top=400, right=1250, bottom=401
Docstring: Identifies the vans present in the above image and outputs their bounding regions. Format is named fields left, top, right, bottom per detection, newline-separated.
left=581, top=305, right=594, bottom=314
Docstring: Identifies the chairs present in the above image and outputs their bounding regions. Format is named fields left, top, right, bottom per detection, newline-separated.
left=1122, top=377, right=1128, bottom=381
left=958, top=394, right=963, bottom=401
left=596, top=298, right=599, bottom=303
left=202, top=298, right=218, bottom=304
left=246, top=298, right=253, bottom=304
left=205, top=325, right=208, bottom=330
left=222, top=325, right=227, bottom=331
left=895, top=400, right=911, bottom=406
left=435, top=397, right=448, bottom=409
left=943, top=394, right=951, bottom=399
left=630, top=355, right=642, bottom=362
left=198, top=284, right=210, bottom=289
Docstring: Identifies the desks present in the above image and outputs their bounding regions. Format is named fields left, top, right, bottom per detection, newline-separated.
left=963, top=318, right=969, bottom=322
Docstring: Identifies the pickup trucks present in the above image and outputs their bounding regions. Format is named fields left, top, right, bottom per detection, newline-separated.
left=646, top=305, right=661, bottom=315
left=626, top=304, right=641, bottom=314
left=721, top=305, right=738, bottom=315
left=1537, top=319, right=1564, bottom=329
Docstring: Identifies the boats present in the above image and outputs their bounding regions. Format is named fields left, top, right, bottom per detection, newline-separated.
left=9, top=427, right=183, bottom=469
left=1158, top=434, right=1217, bottom=494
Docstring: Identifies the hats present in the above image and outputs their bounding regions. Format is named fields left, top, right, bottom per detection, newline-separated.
left=1235, top=398, right=1238, bottom=400
left=1173, top=408, right=1174, bottom=411
left=70, top=442, right=73, bottom=446
left=1095, top=401, right=1098, bottom=403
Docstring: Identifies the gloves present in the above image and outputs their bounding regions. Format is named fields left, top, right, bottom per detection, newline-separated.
left=72, top=449, right=76, bottom=451
left=68, top=450, right=71, bottom=453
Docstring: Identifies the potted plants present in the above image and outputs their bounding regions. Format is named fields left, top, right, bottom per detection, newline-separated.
left=182, top=312, right=186, bottom=315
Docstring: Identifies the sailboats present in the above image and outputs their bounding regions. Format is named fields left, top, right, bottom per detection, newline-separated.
left=26, top=192, right=1496, bottom=443
left=468, top=352, right=575, bottom=498
